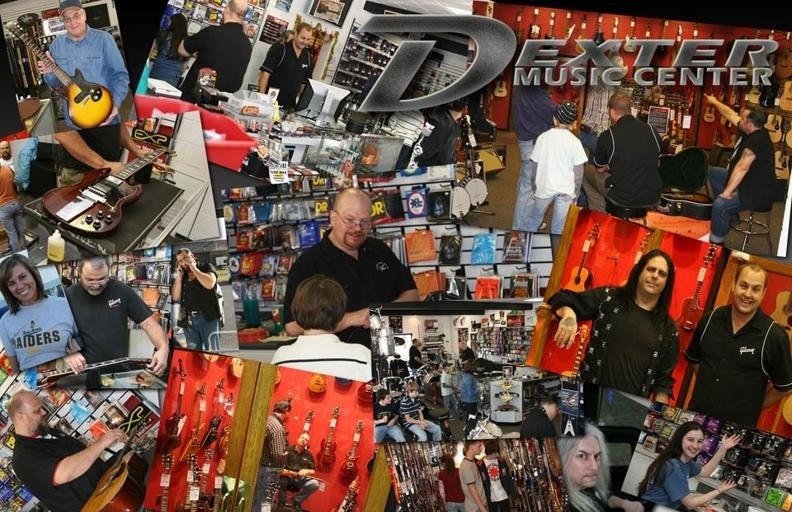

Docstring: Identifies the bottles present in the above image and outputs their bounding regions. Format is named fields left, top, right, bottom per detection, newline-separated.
left=305, top=146, right=360, bottom=184
left=46, top=228, right=64, bottom=261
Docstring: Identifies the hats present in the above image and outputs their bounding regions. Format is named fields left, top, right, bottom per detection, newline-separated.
left=555, top=99, right=578, bottom=124
left=59, top=0, right=83, bottom=15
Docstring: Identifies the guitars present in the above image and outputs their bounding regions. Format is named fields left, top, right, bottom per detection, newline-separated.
left=217, top=393, right=237, bottom=458
left=78, top=418, right=151, bottom=512
left=4, top=19, right=113, bottom=130
left=673, top=243, right=719, bottom=353
left=563, top=220, right=602, bottom=294
left=357, top=382, right=374, bottom=403
left=202, top=378, right=227, bottom=451
left=41, top=144, right=177, bottom=237
left=165, top=359, right=191, bottom=443
left=228, top=357, right=244, bottom=379
left=210, top=458, right=228, bottom=511
left=156, top=453, right=173, bottom=512
left=185, top=449, right=213, bottom=512
left=768, top=279, right=791, bottom=346
left=316, top=406, right=343, bottom=465
left=308, top=372, right=328, bottom=394
left=340, top=418, right=364, bottom=477
left=181, top=381, right=210, bottom=463
left=702, top=78, right=791, bottom=183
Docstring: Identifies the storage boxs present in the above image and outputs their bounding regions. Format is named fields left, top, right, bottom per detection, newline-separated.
left=135, top=94, right=258, bottom=173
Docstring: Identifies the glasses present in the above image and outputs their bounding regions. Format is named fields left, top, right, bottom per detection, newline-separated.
left=86, top=279, right=105, bottom=285
left=65, top=11, right=83, bottom=24
left=175, top=249, right=189, bottom=255
left=333, top=208, right=371, bottom=229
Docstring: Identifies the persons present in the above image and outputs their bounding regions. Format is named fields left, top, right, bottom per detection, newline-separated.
left=637, top=421, right=742, bottom=512
left=682, top=262, right=791, bottom=430
left=250, top=402, right=322, bottom=512
left=271, top=273, right=374, bottom=382
left=521, top=395, right=560, bottom=439
left=65, top=254, right=170, bottom=378
left=283, top=189, right=419, bottom=347
left=0, top=126, right=151, bottom=252
left=514, top=57, right=662, bottom=238
left=167, top=245, right=224, bottom=355
left=702, top=93, right=776, bottom=247
left=257, top=21, right=315, bottom=120
left=555, top=420, right=646, bottom=512
left=545, top=249, right=680, bottom=407
left=36, top=1, right=130, bottom=131
left=374, top=338, right=481, bottom=442
left=146, top=1, right=252, bottom=114
left=1, top=254, right=85, bottom=375
left=345, top=69, right=468, bottom=166
left=6, top=390, right=128, bottom=511
left=437, top=440, right=521, bottom=512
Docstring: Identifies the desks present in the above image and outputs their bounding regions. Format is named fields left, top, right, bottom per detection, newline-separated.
left=22, top=162, right=209, bottom=255
left=247, top=120, right=359, bottom=163
left=25, top=99, right=59, bottom=137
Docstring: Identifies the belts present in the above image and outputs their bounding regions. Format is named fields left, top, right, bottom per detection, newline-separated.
left=187, top=310, right=203, bottom=316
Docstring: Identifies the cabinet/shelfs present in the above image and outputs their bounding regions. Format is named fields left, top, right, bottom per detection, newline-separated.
left=489, top=375, right=561, bottom=423
left=620, top=411, right=785, bottom=512
left=469, top=324, right=535, bottom=364
left=124, top=260, right=172, bottom=334
left=220, top=180, right=554, bottom=336
left=333, top=23, right=399, bottom=102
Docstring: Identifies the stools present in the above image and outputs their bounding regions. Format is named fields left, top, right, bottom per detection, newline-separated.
left=605, top=200, right=647, bottom=225
left=428, top=408, right=453, bottom=441
left=730, top=210, right=772, bottom=255
left=281, top=485, right=306, bottom=511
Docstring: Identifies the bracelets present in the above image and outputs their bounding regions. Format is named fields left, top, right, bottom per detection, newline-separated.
left=716, top=487, right=724, bottom=497
